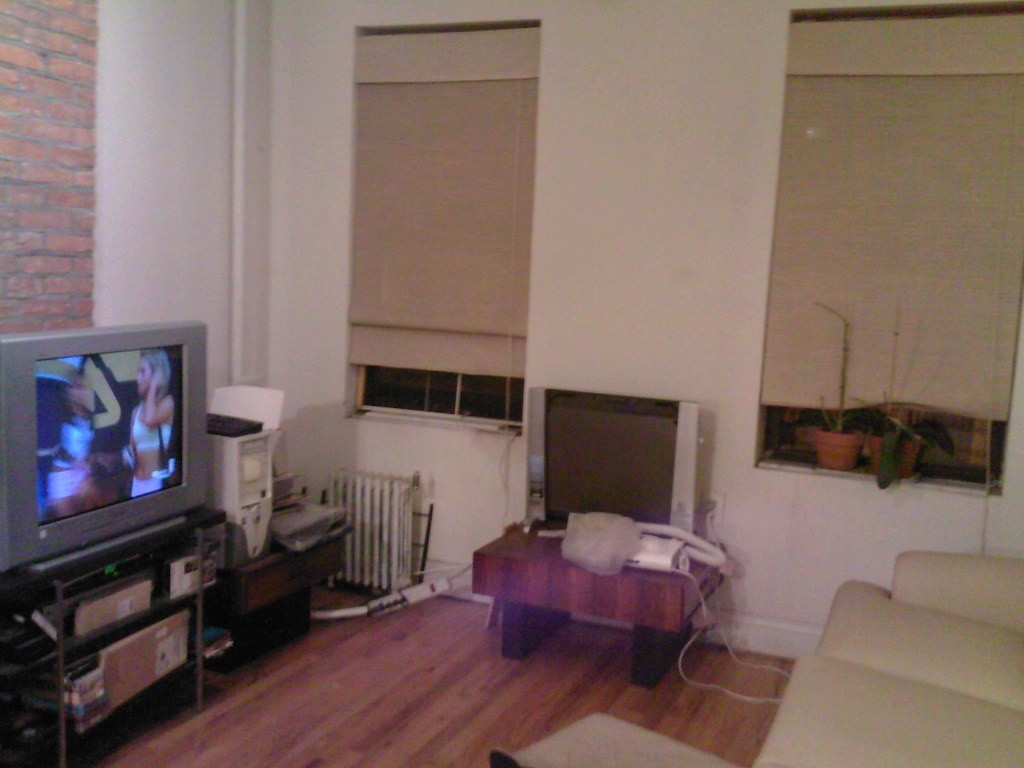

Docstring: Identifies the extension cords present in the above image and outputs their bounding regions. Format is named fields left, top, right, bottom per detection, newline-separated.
left=367, top=577, right=454, bottom=617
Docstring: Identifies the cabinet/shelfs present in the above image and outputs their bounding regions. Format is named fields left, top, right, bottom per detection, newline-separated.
left=0, top=505, right=353, bottom=768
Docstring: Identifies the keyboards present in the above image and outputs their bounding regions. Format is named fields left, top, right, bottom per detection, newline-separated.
left=207, top=414, right=263, bottom=438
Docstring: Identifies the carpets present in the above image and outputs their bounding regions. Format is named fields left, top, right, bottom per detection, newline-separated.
left=510, top=711, right=745, bottom=768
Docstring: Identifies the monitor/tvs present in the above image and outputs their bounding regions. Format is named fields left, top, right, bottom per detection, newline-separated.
left=0, top=321, right=208, bottom=578
left=523, top=388, right=699, bottom=541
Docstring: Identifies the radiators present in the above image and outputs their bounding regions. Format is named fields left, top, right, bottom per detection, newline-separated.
left=325, top=469, right=419, bottom=594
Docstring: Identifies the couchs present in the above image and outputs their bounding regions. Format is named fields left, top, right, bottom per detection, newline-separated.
left=752, top=549, right=1024, bottom=768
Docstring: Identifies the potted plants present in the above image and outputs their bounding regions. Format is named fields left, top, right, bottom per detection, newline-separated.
left=810, top=298, right=956, bottom=491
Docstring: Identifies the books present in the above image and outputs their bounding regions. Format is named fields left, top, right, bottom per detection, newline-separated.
left=201, top=626, right=233, bottom=658
left=273, top=472, right=305, bottom=516
left=22, top=663, right=106, bottom=733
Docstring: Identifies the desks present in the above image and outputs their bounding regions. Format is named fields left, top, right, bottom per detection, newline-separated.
left=471, top=518, right=728, bottom=687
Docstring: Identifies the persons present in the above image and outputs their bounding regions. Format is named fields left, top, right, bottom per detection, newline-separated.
left=48, top=389, right=102, bottom=516
left=123, top=349, right=173, bottom=497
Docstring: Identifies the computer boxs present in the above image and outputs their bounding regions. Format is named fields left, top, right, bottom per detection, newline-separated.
left=205, top=429, right=273, bottom=570
